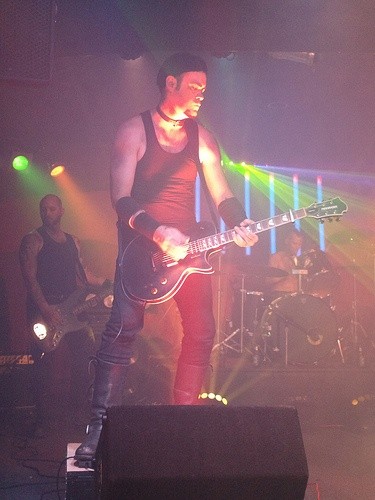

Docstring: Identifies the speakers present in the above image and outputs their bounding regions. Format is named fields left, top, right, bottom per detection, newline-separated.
left=97, top=405, right=308, bottom=500
left=0, top=354, right=38, bottom=411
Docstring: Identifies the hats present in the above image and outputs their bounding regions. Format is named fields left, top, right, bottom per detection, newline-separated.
left=156, top=53, right=208, bottom=89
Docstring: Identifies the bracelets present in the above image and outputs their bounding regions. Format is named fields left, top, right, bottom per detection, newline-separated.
left=133, top=209, right=161, bottom=241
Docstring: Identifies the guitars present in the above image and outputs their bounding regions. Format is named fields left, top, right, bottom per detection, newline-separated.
left=121, top=195, right=350, bottom=304
left=28, top=285, right=116, bottom=351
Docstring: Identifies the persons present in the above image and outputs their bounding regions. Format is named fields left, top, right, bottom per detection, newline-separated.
left=265, top=230, right=306, bottom=293
left=18, top=194, right=96, bottom=437
left=74, top=51, right=258, bottom=459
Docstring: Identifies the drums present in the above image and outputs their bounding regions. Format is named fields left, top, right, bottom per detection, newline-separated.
left=230, top=287, right=264, bottom=335
left=296, top=248, right=341, bottom=297
left=252, top=290, right=338, bottom=367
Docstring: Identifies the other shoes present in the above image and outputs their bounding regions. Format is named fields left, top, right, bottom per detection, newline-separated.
left=33, top=420, right=48, bottom=436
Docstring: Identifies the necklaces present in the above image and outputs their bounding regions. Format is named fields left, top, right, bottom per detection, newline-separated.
left=156, top=104, right=186, bottom=128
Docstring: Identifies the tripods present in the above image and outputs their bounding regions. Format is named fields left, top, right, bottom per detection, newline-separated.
left=213, top=275, right=254, bottom=353
left=347, top=272, right=367, bottom=348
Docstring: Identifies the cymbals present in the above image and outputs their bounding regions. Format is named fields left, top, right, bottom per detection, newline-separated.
left=234, top=263, right=290, bottom=278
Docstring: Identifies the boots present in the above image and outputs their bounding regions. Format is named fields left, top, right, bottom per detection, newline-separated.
left=73, top=355, right=129, bottom=460
left=172, top=363, right=208, bottom=406
